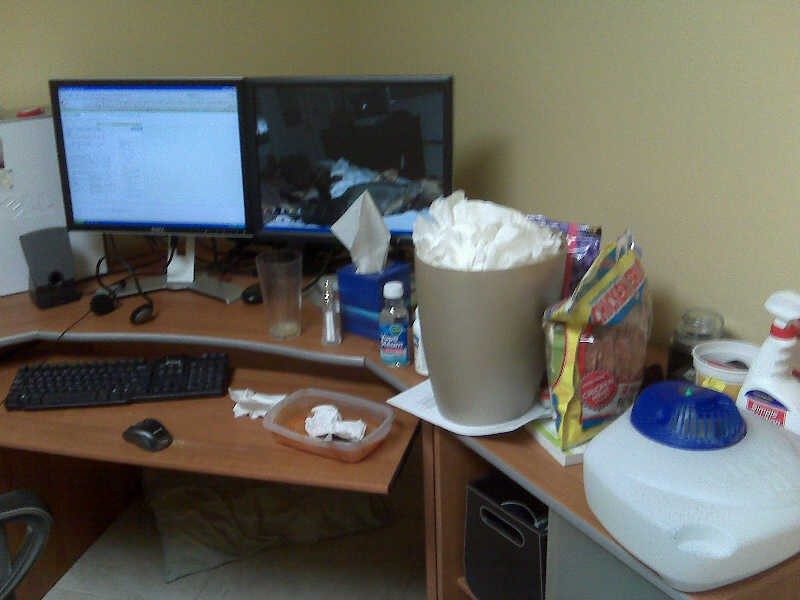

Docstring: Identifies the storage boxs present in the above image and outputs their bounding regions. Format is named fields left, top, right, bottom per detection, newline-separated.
left=463, top=478, right=548, bottom=600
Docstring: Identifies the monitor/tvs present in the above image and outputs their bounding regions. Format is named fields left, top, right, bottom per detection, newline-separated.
left=252, top=75, right=453, bottom=304
left=48, top=76, right=259, bottom=303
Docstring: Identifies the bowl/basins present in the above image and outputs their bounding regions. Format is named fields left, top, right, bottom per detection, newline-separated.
left=264, top=388, right=394, bottom=463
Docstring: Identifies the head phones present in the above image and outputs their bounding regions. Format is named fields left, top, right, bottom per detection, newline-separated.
left=91, top=255, right=153, bottom=325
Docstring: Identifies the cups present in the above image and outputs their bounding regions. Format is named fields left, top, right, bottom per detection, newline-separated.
left=256, top=249, right=303, bottom=339
left=692, top=341, right=758, bottom=403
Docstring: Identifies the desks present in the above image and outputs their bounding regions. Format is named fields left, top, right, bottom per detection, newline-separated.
left=0, top=260, right=800, bottom=600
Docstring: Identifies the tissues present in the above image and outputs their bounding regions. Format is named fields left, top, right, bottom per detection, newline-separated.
left=331, top=189, right=411, bottom=341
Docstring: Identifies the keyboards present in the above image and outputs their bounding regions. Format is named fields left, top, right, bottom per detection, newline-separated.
left=3, top=354, right=229, bottom=410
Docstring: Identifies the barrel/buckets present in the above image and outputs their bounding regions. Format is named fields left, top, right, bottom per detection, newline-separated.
left=413, top=229, right=567, bottom=427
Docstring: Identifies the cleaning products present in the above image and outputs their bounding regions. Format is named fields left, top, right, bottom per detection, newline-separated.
left=735, top=288, right=800, bottom=434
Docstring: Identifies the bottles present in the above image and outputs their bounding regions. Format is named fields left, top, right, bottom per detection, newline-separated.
left=379, top=281, right=410, bottom=368
left=667, top=309, right=724, bottom=383
left=321, top=278, right=342, bottom=345
left=413, top=307, right=429, bottom=376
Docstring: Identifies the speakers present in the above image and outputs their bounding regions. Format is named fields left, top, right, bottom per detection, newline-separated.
left=19, top=227, right=79, bottom=308
left=462, top=475, right=548, bottom=600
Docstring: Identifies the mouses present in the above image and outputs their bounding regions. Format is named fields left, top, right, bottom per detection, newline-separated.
left=241, top=282, right=261, bottom=304
left=123, top=419, right=172, bottom=451
